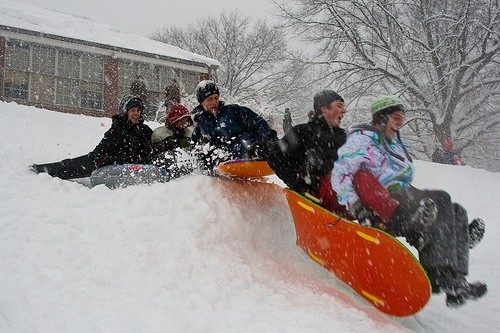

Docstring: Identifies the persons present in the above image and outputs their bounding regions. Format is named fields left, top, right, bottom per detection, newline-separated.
left=268, top=88, right=486, bottom=253
left=30, top=88, right=153, bottom=180
left=190, top=79, right=279, bottom=176
left=129, top=74, right=148, bottom=105
left=164, top=77, right=181, bottom=112
left=152, top=103, right=195, bottom=167
left=331, top=95, right=487, bottom=308
left=432, top=136, right=465, bottom=166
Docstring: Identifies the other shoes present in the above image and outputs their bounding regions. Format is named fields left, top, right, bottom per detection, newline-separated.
left=400, top=197, right=438, bottom=251
left=469, top=218, right=486, bottom=249
left=427, top=267, right=470, bottom=296
left=446, top=281, right=487, bottom=307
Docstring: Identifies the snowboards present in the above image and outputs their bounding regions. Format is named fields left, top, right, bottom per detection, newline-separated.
left=216, top=157, right=282, bottom=181
left=282, top=188, right=432, bottom=320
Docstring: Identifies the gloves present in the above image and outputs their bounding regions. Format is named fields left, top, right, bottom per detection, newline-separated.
left=347, top=198, right=375, bottom=227
left=282, top=107, right=300, bottom=147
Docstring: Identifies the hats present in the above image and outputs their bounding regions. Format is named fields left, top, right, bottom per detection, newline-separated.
left=125, top=99, right=142, bottom=112
left=195, top=80, right=220, bottom=104
left=166, top=104, right=194, bottom=129
left=370, top=95, right=405, bottom=116
left=313, top=89, right=344, bottom=114
